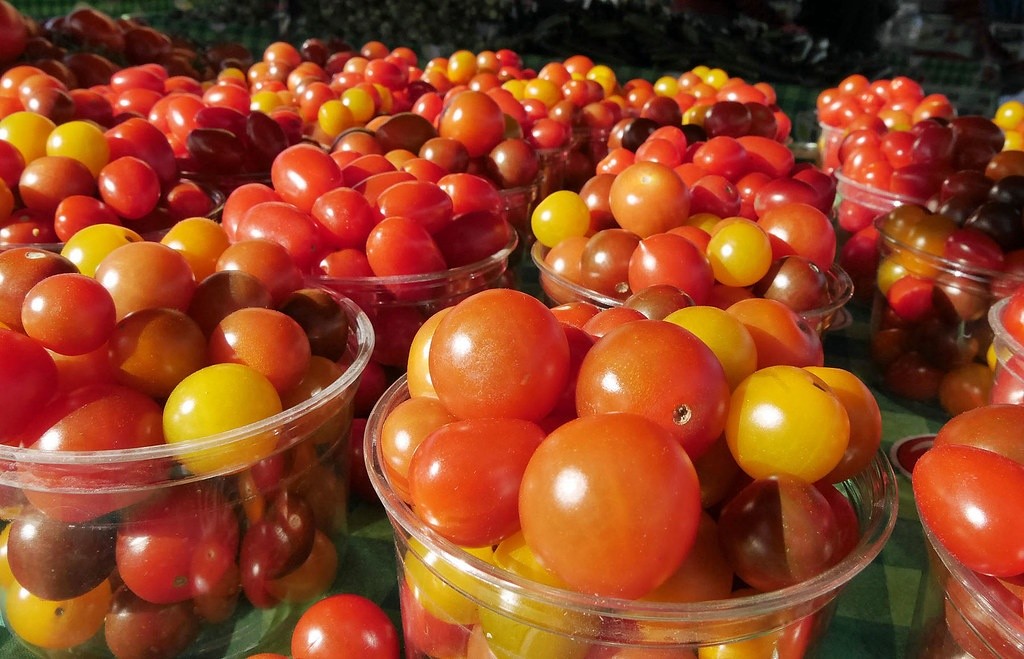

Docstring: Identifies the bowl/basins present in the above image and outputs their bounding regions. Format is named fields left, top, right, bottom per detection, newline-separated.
left=1, top=164, right=1023, bottom=659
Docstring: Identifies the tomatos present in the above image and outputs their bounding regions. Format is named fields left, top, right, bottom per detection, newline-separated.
left=0, top=0, right=1024, bottom=659
